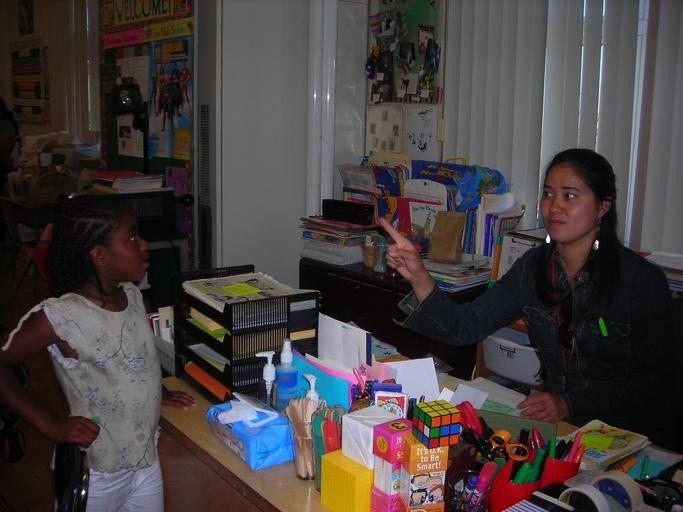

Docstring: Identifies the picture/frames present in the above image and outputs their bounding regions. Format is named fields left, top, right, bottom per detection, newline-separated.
left=158, top=320, right=683, bottom=511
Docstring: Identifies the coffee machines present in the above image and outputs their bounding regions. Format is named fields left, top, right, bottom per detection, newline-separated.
left=456, top=400, right=483, bottom=438
left=480, top=429, right=530, bottom=463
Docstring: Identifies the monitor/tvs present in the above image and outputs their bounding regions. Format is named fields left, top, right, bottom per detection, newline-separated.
left=553, top=418, right=649, bottom=470
left=398, top=180, right=461, bottom=242
left=142, top=306, right=175, bottom=340
left=184, top=271, right=318, bottom=312
left=463, top=190, right=527, bottom=260
left=644, top=251, right=682, bottom=302
left=298, top=214, right=386, bottom=267
left=78, top=169, right=174, bottom=194
left=417, top=259, right=492, bottom=293
left=336, top=151, right=409, bottom=197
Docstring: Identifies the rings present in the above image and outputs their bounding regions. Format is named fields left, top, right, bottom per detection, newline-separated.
left=351, top=365, right=378, bottom=400
left=639, top=454, right=651, bottom=483
left=599, top=319, right=609, bottom=337
left=407, top=394, right=425, bottom=422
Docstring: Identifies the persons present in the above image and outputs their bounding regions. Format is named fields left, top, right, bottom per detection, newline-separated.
left=0, top=196, right=196, bottom=512
left=0, top=97, right=21, bottom=197
left=380, top=149, right=682, bottom=455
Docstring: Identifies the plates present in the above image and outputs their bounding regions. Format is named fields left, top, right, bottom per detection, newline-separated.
left=298, top=253, right=490, bottom=388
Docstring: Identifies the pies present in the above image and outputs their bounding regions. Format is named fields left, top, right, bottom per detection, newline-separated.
left=556, top=439, right=565, bottom=462
left=504, top=427, right=544, bottom=486
left=548, top=436, right=556, bottom=461
left=569, top=431, right=584, bottom=463
left=574, top=443, right=587, bottom=466
left=560, top=439, right=573, bottom=460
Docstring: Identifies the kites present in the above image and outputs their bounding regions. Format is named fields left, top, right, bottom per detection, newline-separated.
left=461, top=475, right=478, bottom=501
left=470, top=462, right=498, bottom=508
